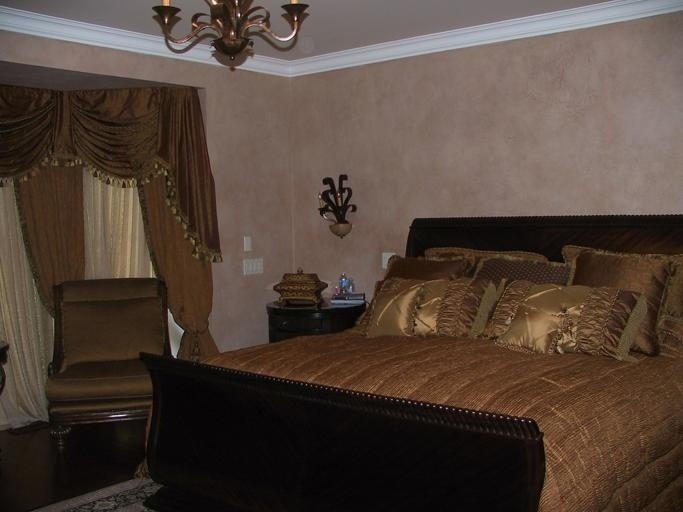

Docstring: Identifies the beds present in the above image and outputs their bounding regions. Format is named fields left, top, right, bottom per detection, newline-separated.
left=140, top=216, right=683, bottom=512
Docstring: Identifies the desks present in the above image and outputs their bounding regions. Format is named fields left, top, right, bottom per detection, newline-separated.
left=0, top=341, right=10, bottom=394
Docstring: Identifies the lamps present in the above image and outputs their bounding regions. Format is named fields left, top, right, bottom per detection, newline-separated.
left=151, top=0, right=310, bottom=60
left=317, top=175, right=357, bottom=239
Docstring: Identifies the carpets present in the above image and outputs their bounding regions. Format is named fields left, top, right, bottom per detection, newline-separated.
left=26, top=475, right=164, bottom=512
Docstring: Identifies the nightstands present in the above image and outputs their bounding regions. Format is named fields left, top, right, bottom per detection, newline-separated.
left=266, top=304, right=366, bottom=342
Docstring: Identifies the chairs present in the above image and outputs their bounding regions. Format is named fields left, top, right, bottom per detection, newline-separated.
left=45, top=278, right=168, bottom=451
